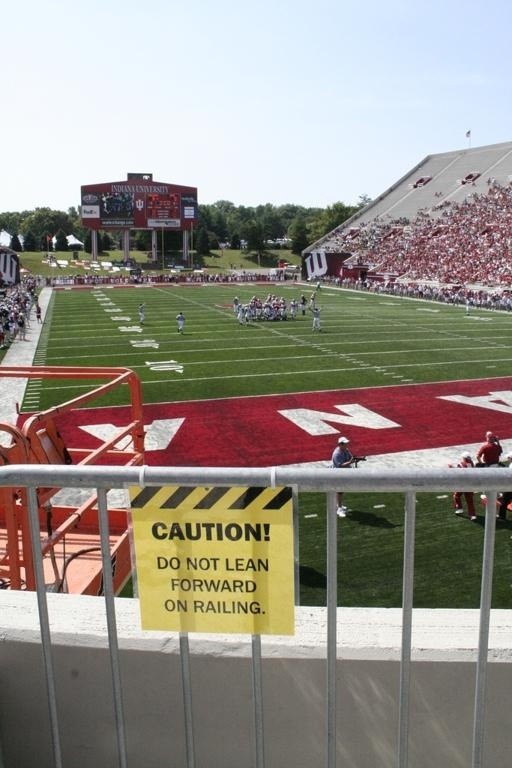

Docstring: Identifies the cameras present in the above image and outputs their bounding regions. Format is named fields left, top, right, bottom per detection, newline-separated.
left=353, top=455, right=367, bottom=462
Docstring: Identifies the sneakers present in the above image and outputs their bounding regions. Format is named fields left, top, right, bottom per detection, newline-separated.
left=471, top=516, right=477, bottom=521
left=337, top=504, right=347, bottom=518
left=455, top=509, right=464, bottom=513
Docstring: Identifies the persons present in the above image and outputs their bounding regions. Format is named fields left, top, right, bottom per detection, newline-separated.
left=0, top=272, right=44, bottom=350
left=447, top=453, right=479, bottom=521
left=303, top=171, right=512, bottom=312
left=233, top=292, right=288, bottom=326
left=476, top=430, right=504, bottom=468
left=299, top=293, right=308, bottom=315
left=289, top=298, right=298, bottom=320
left=496, top=451, right=512, bottom=519
left=308, top=304, right=324, bottom=331
left=329, top=435, right=359, bottom=519
left=46, top=268, right=302, bottom=288
left=138, top=300, right=147, bottom=324
left=175, top=311, right=186, bottom=335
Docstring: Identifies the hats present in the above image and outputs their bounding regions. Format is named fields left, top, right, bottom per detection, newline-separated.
left=338, top=437, right=350, bottom=444
left=485, top=431, right=494, bottom=438
left=461, top=451, right=471, bottom=459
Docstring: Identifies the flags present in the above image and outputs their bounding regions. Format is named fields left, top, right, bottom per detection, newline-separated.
left=466, top=129, right=471, bottom=139
left=47, top=233, right=52, bottom=241
